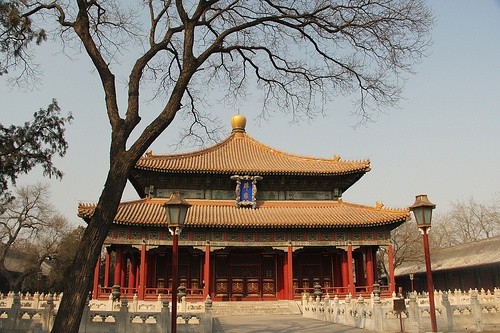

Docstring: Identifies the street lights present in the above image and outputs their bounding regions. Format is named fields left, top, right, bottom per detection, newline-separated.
left=162, top=191, right=193, bottom=332
left=408, top=271, right=414, bottom=292
left=37, top=256, right=52, bottom=285
left=408, top=194, right=438, bottom=333
